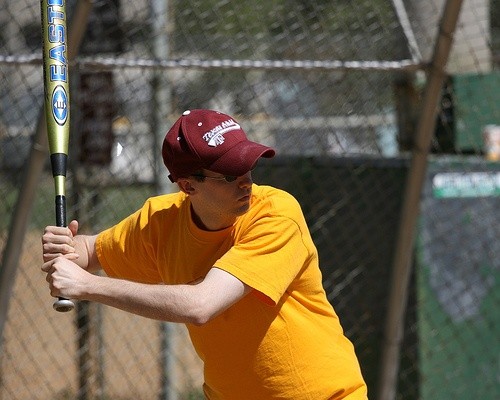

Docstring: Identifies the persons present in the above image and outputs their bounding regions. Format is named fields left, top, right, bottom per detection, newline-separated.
left=41, top=109, right=369, bottom=400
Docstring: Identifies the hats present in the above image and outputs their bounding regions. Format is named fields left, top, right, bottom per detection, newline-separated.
left=161, top=109, right=275, bottom=183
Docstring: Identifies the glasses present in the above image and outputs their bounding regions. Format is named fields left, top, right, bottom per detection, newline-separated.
left=178, top=157, right=259, bottom=183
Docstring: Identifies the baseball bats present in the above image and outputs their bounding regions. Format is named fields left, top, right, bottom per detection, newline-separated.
left=40, top=0, right=75, bottom=313
